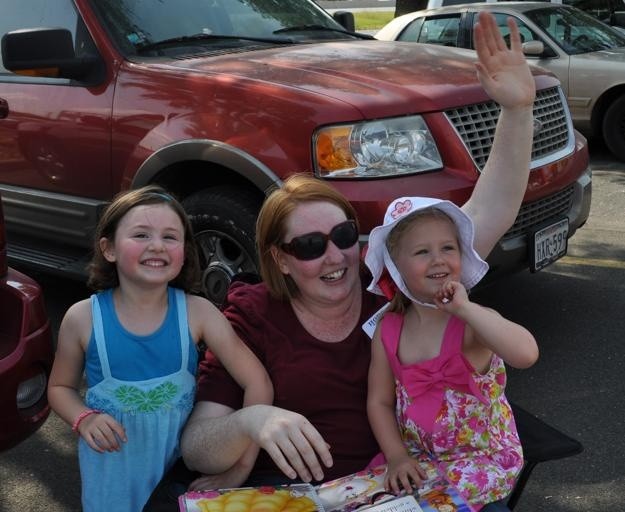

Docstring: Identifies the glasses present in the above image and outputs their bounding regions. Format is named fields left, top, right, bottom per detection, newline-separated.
left=276, top=217, right=361, bottom=261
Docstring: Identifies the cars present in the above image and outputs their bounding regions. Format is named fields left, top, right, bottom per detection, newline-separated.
left=355, top=0, right=625, bottom=162
left=0, top=266, right=55, bottom=452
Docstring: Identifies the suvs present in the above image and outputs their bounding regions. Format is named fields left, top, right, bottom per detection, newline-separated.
left=0, top=0, right=593, bottom=361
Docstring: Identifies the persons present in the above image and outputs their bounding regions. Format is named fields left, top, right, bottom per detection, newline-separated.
left=362, top=194, right=543, bottom=512
left=45, top=184, right=278, bottom=512
left=176, top=10, right=538, bottom=512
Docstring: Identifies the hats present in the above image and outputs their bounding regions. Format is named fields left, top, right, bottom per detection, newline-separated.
left=363, top=192, right=491, bottom=305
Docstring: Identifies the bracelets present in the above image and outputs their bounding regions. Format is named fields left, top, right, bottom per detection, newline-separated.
left=71, top=407, right=102, bottom=432
